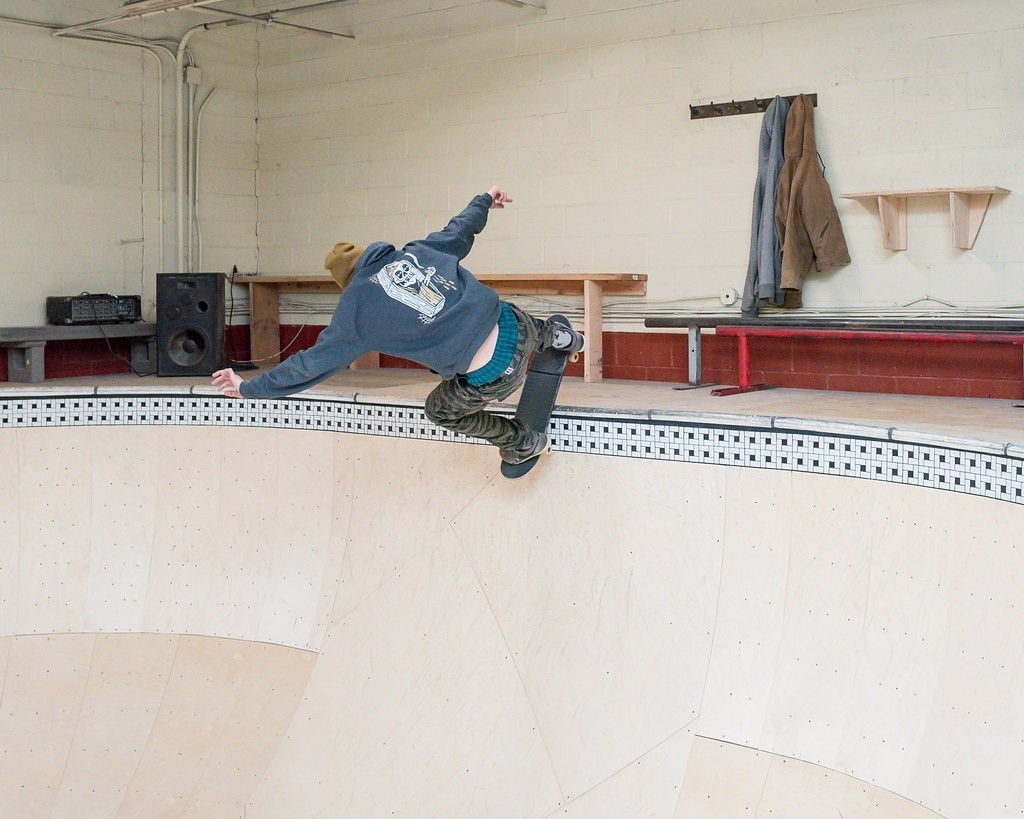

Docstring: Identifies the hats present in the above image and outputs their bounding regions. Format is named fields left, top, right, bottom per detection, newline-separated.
left=324, top=242, right=365, bottom=288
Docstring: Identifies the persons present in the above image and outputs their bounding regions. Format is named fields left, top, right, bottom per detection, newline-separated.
left=210, top=186, right=588, bottom=464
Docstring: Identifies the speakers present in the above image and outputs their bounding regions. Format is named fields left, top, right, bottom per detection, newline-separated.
left=155, top=272, right=226, bottom=377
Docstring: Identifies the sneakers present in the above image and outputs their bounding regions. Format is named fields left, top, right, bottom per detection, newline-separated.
left=546, top=320, right=588, bottom=352
left=498, top=430, right=552, bottom=463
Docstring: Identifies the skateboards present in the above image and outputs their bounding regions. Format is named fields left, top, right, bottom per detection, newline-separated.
left=498, top=310, right=574, bottom=478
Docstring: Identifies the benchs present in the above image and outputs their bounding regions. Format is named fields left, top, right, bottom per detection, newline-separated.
left=1, top=320, right=161, bottom=388
left=637, top=310, right=1023, bottom=409
left=230, top=268, right=649, bottom=384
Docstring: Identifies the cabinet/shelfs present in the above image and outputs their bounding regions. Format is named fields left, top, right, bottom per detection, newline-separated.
left=837, top=184, right=1012, bottom=251
